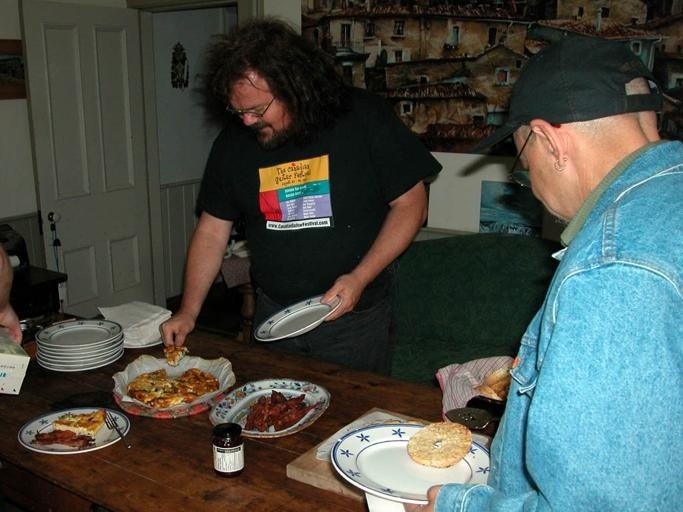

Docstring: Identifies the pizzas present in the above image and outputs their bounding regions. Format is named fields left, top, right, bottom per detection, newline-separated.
left=53, top=409, right=106, bottom=437
left=163, top=346, right=188, bottom=367
left=127, top=368, right=219, bottom=408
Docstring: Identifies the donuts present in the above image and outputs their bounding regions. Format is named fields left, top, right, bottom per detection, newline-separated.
left=407, top=421, right=474, bottom=468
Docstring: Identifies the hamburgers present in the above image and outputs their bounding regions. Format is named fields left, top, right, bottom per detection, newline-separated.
left=477, top=367, right=511, bottom=401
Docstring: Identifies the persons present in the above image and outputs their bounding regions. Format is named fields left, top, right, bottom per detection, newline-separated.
left=402, top=31, right=683, bottom=512
left=158, top=17, right=443, bottom=377
left=0, top=243, right=23, bottom=344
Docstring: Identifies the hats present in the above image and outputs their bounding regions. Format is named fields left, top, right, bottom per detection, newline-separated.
left=470, top=37, right=664, bottom=153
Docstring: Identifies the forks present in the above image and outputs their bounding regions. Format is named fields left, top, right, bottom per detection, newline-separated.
left=102, top=412, right=135, bottom=451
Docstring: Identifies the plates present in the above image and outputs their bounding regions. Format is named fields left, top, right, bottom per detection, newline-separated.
left=112, top=353, right=233, bottom=420
left=210, top=376, right=333, bottom=442
left=17, top=403, right=132, bottom=457
left=333, top=422, right=493, bottom=508
left=122, top=334, right=167, bottom=351
left=34, top=319, right=127, bottom=373
left=251, top=291, right=342, bottom=343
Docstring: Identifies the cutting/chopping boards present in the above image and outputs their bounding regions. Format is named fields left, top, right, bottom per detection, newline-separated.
left=286, top=406, right=493, bottom=504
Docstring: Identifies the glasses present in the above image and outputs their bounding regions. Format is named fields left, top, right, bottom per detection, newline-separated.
left=508, top=124, right=562, bottom=190
left=226, top=95, right=276, bottom=118
